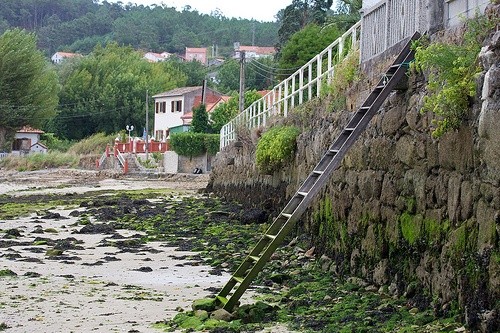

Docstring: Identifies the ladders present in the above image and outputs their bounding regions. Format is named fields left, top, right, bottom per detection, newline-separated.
left=211, top=30, right=428, bottom=315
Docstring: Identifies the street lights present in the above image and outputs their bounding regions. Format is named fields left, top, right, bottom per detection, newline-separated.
left=126, top=125, right=134, bottom=143
